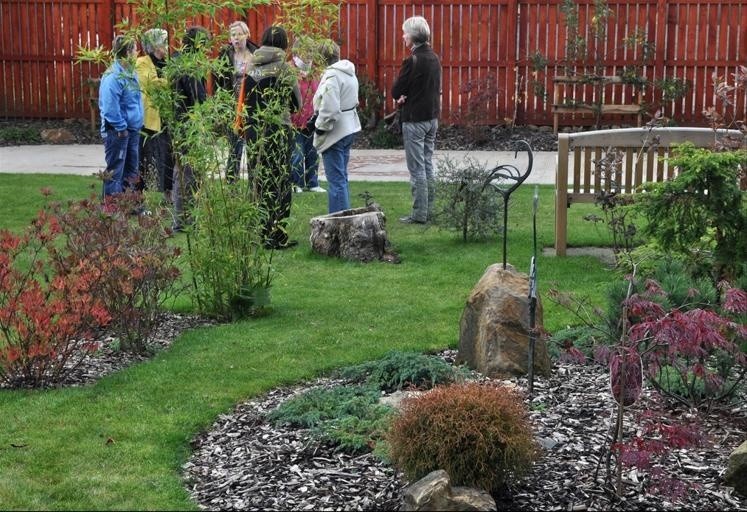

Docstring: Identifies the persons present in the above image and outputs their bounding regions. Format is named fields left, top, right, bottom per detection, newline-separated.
left=241, top=26, right=299, bottom=248
left=313, top=38, right=363, bottom=212
left=133, top=29, right=171, bottom=192
left=166, top=27, right=213, bottom=234
left=98, top=36, right=146, bottom=214
left=392, top=16, right=442, bottom=223
left=213, top=20, right=264, bottom=189
left=286, top=38, right=328, bottom=193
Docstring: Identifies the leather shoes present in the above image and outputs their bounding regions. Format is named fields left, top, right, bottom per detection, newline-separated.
left=264, top=239, right=299, bottom=250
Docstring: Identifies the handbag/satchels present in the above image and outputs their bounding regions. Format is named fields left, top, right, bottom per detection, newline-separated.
left=383, top=109, right=402, bottom=137
left=306, top=114, right=318, bottom=132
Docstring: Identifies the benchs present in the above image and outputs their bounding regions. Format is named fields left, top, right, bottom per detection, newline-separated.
left=555, top=126, right=746, bottom=257
left=550, top=76, right=642, bottom=152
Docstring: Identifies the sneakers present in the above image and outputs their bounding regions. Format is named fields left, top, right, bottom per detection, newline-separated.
left=139, top=209, right=152, bottom=216
left=399, top=215, right=426, bottom=225
left=292, top=182, right=327, bottom=193
